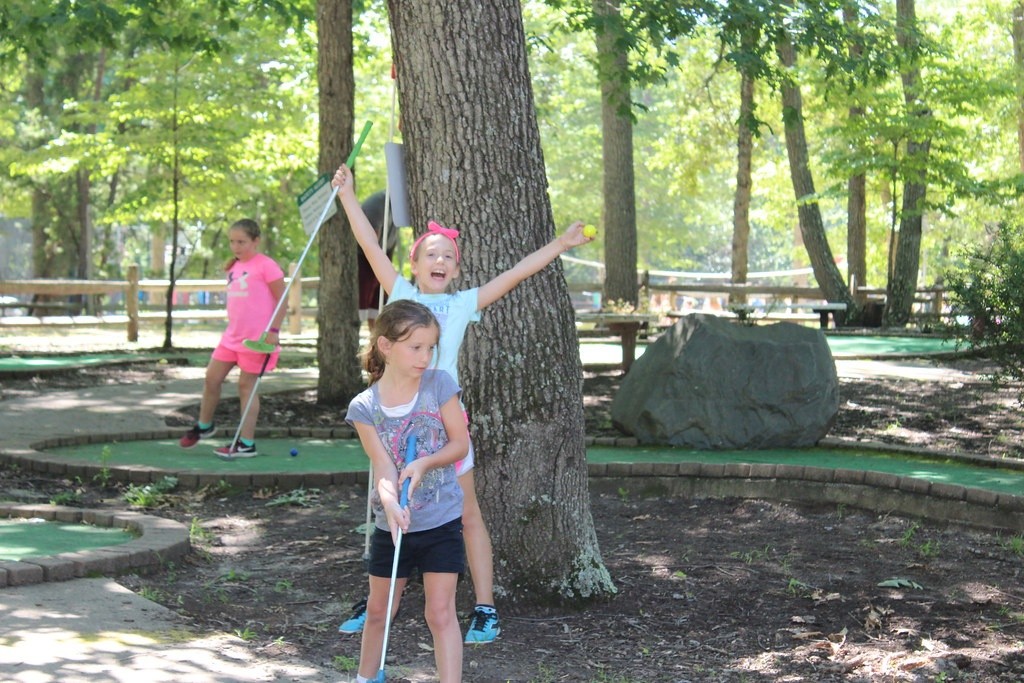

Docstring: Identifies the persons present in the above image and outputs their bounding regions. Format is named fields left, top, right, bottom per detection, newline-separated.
left=180, top=218, right=289, bottom=459
left=333, top=163, right=598, bottom=644
left=343, top=299, right=470, bottom=682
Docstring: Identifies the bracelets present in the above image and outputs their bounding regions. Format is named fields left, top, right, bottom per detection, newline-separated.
left=269, top=328, right=279, bottom=333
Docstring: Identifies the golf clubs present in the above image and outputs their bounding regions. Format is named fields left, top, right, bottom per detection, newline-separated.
left=365, top=430, right=419, bottom=683
left=241, top=117, right=376, bottom=355
left=218, top=352, right=271, bottom=462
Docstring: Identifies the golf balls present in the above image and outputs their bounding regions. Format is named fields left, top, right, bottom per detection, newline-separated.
left=582, top=224, right=597, bottom=238
left=289, top=447, right=298, bottom=457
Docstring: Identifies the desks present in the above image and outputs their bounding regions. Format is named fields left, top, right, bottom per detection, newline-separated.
left=727, top=302, right=847, bottom=327
left=575, top=312, right=659, bottom=371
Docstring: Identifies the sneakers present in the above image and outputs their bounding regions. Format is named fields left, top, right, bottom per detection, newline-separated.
left=339, top=597, right=366, bottom=633
left=213, top=439, right=257, bottom=457
left=179, top=422, right=216, bottom=449
left=464, top=604, right=501, bottom=644
